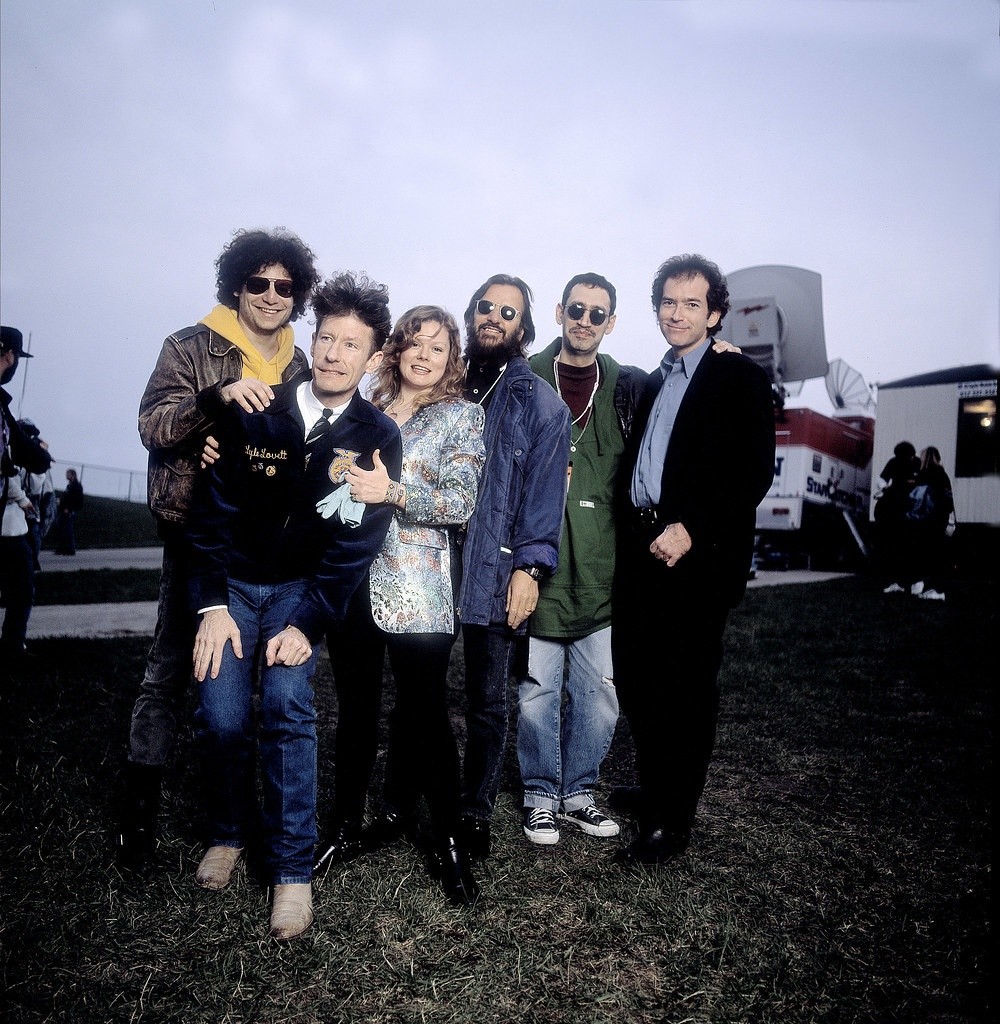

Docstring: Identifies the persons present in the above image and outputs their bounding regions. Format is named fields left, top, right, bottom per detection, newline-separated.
left=448, top=273, right=571, bottom=859
left=613, top=255, right=775, bottom=869
left=183, top=274, right=403, bottom=938
left=123, top=234, right=316, bottom=861
left=517, top=272, right=745, bottom=846
left=0, top=325, right=84, bottom=687
left=872, top=440, right=956, bottom=601
left=322, top=303, right=484, bottom=910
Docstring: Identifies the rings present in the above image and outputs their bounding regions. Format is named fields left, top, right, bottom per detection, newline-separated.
left=526, top=608, right=533, bottom=612
left=662, top=555, right=669, bottom=561
left=351, top=494, right=358, bottom=501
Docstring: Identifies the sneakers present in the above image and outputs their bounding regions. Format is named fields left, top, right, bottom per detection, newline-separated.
left=555, top=804, right=619, bottom=837
left=523, top=806, right=559, bottom=844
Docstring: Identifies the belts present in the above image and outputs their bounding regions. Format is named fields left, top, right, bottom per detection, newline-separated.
left=635, top=505, right=659, bottom=528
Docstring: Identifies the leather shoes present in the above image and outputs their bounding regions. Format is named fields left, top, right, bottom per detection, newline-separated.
left=361, top=808, right=418, bottom=846
left=615, top=825, right=687, bottom=865
left=429, top=835, right=480, bottom=906
left=464, top=815, right=490, bottom=858
left=610, top=785, right=649, bottom=814
left=311, top=821, right=368, bottom=875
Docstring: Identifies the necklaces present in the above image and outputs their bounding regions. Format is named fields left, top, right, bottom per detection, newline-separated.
left=387, top=404, right=411, bottom=419
left=463, top=356, right=508, bottom=407
left=552, top=350, right=600, bottom=448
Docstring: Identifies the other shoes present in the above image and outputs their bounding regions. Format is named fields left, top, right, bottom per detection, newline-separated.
left=910, top=581, right=924, bottom=595
left=270, top=881, right=314, bottom=942
left=883, top=583, right=905, bottom=593
left=193, top=845, right=241, bottom=892
left=918, top=588, right=946, bottom=601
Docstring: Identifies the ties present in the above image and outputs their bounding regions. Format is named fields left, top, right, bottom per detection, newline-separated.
left=304, top=408, right=333, bottom=472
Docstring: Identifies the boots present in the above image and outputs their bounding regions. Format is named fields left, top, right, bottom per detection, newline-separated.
left=115, top=760, right=162, bottom=873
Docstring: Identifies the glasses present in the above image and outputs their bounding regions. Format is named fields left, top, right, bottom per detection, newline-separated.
left=475, top=299, right=523, bottom=321
left=242, top=276, right=295, bottom=299
left=564, top=304, right=610, bottom=327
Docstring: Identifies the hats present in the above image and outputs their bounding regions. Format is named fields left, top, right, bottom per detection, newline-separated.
left=0, top=326, right=33, bottom=357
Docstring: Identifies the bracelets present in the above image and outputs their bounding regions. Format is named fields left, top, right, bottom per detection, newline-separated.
left=523, top=566, right=543, bottom=582
left=384, top=480, right=404, bottom=504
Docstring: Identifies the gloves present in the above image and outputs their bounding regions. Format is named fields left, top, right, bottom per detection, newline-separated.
left=316, top=472, right=366, bottom=529
